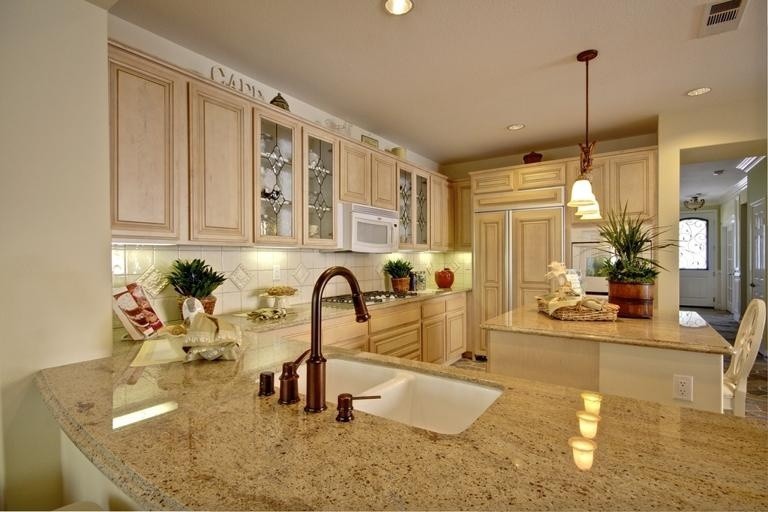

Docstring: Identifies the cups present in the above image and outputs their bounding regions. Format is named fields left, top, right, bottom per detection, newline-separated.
left=309, top=150, right=319, bottom=166
left=309, top=224, right=320, bottom=236
left=262, top=214, right=273, bottom=235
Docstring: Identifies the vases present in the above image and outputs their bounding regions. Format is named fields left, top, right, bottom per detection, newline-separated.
left=435, top=268, right=455, bottom=288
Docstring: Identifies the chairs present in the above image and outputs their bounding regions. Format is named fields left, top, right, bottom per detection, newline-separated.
left=723, top=298, right=767, bottom=416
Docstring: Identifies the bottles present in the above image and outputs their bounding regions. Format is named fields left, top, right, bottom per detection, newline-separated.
left=416, top=270, right=426, bottom=290
left=410, top=272, right=416, bottom=291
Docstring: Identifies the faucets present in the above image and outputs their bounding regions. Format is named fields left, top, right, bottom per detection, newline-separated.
left=304, top=265, right=371, bottom=414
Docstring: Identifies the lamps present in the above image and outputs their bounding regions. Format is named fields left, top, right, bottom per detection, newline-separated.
left=684, top=197, right=705, bottom=210
left=383, top=0, right=414, bottom=16
left=576, top=201, right=599, bottom=214
left=581, top=213, right=602, bottom=220
left=569, top=389, right=603, bottom=472
left=567, top=50, right=598, bottom=207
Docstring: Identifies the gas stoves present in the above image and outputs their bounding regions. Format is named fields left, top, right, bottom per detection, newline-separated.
left=319, top=289, right=410, bottom=304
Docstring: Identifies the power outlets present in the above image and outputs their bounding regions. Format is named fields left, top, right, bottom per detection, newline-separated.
left=673, top=373, right=692, bottom=402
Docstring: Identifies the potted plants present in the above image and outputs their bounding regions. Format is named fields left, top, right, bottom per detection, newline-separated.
left=590, top=201, right=680, bottom=319
left=384, top=260, right=414, bottom=291
left=166, top=257, right=227, bottom=321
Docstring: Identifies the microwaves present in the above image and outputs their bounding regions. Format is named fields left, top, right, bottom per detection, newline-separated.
left=343, top=204, right=401, bottom=253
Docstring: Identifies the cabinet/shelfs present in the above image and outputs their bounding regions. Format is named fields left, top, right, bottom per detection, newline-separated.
left=430, top=169, right=454, bottom=250
left=108, top=36, right=248, bottom=248
left=249, top=97, right=340, bottom=250
left=257, top=293, right=467, bottom=368
left=340, top=135, right=399, bottom=210
left=399, top=159, right=429, bottom=248
left=471, top=159, right=563, bottom=364
left=564, top=146, right=656, bottom=227
left=454, top=177, right=471, bottom=252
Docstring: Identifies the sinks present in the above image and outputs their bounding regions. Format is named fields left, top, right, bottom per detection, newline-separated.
left=250, top=356, right=399, bottom=407
left=347, top=371, right=506, bottom=436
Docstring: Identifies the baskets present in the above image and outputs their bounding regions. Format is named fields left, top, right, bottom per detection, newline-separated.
left=534, top=292, right=620, bottom=322
